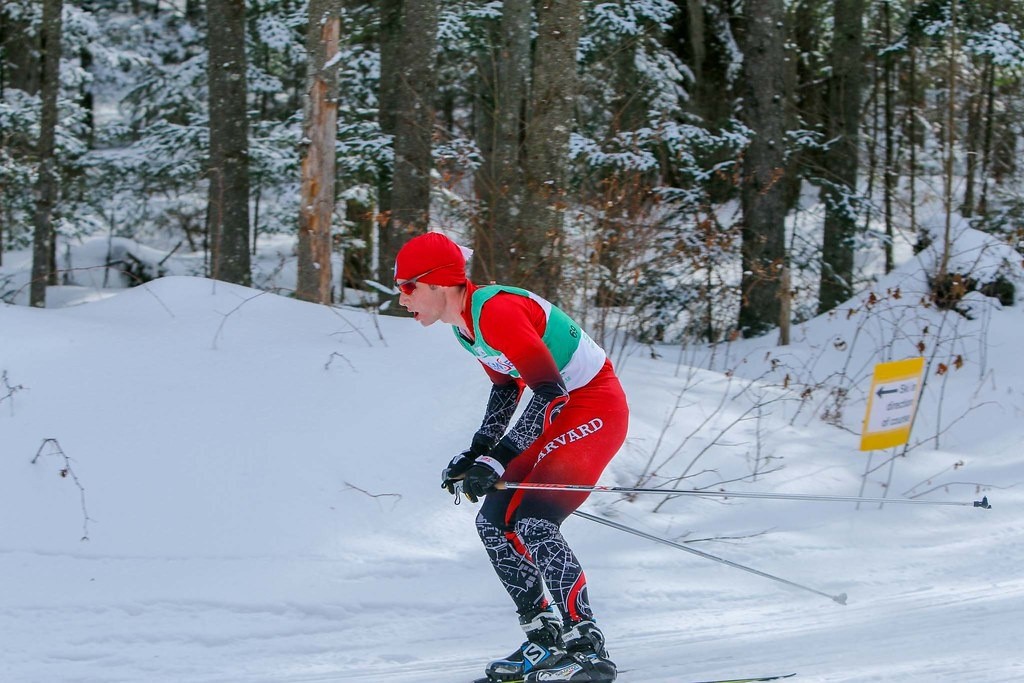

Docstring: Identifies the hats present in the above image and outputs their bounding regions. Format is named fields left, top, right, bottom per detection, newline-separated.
left=393, top=232, right=467, bottom=287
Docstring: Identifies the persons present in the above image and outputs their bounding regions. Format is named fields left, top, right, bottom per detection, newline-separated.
left=394, top=232, right=628, bottom=683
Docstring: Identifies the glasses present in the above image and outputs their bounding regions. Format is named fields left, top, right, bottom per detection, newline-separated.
left=388, top=267, right=438, bottom=297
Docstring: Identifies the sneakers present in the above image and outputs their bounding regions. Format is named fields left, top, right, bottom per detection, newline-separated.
left=484, top=615, right=568, bottom=678
left=525, top=620, right=617, bottom=683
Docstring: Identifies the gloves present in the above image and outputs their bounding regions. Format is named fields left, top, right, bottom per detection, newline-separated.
left=443, top=448, right=483, bottom=495
left=462, top=453, right=508, bottom=503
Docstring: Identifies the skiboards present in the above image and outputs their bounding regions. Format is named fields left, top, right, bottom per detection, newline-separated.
left=475, top=667, right=798, bottom=683
